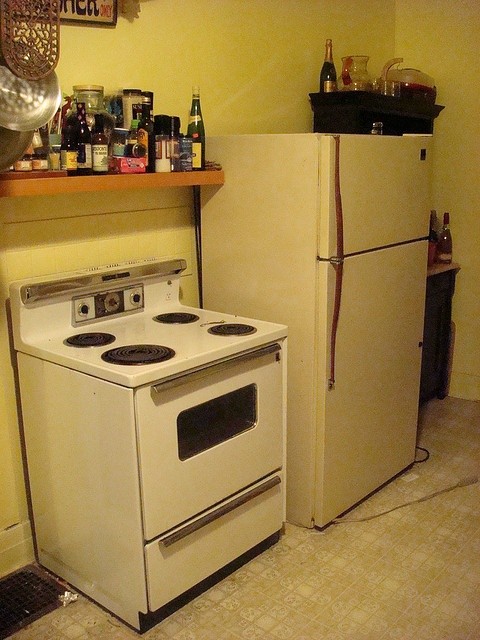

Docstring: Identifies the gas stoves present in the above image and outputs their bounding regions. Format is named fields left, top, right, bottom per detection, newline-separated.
left=64, top=309, right=258, bottom=367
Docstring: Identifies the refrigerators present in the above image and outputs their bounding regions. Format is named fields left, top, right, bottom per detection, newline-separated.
left=197, top=131, right=437, bottom=532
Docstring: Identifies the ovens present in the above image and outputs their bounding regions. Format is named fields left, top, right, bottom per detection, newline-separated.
left=134, top=340, right=288, bottom=615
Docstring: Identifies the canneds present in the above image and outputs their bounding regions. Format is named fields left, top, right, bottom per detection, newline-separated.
left=120, top=87, right=141, bottom=129
left=33, top=153, right=48, bottom=169
left=14, top=154, right=32, bottom=171
left=49, top=144, right=61, bottom=169
left=71, top=84, right=103, bottom=126
left=110, top=126, right=127, bottom=158
left=154, top=133, right=171, bottom=173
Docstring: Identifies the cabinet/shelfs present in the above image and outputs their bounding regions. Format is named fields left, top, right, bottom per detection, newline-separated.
left=308, top=91, right=462, bottom=409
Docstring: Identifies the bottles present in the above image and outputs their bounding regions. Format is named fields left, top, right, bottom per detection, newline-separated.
left=188, top=85, right=206, bottom=171
left=137, top=97, right=156, bottom=173
left=77, top=101, right=92, bottom=175
left=428, top=209, right=438, bottom=263
left=320, top=37, right=336, bottom=91
left=92, top=114, right=109, bottom=175
left=61, top=135, right=77, bottom=175
left=438, top=213, right=452, bottom=264
left=128, top=120, right=140, bottom=144
